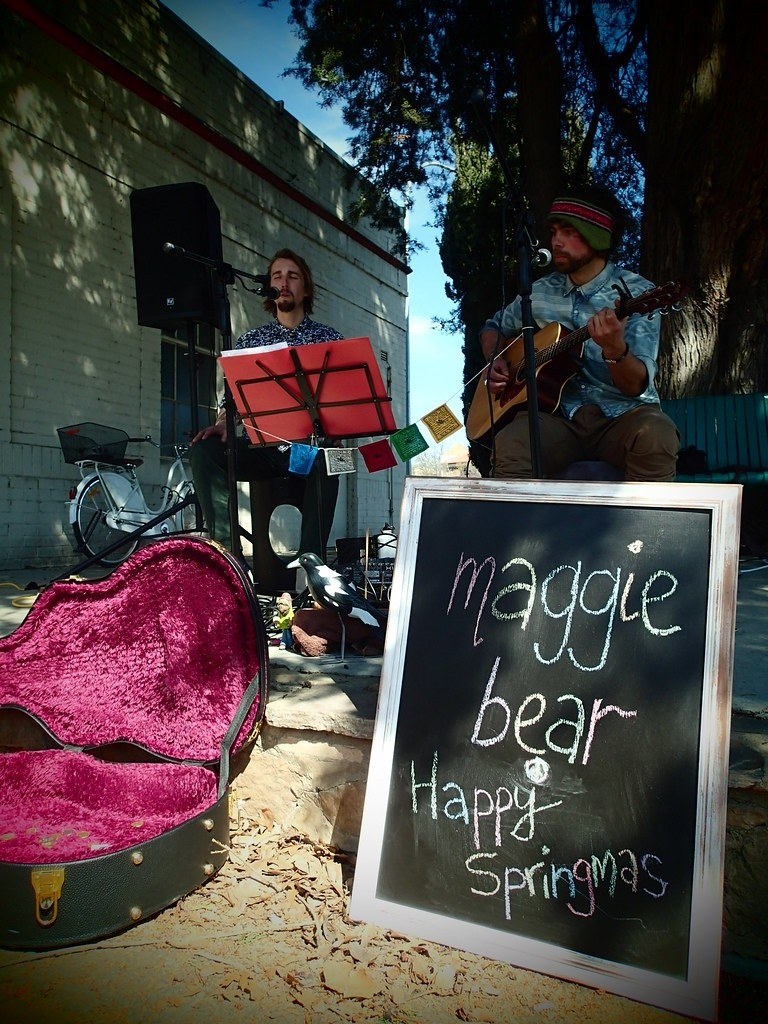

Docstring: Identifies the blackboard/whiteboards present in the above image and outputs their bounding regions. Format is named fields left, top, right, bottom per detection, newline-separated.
left=346, top=476, right=742, bottom=1024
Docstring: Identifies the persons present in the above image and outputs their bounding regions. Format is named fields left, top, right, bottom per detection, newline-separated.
left=480, top=193, right=681, bottom=481
left=190, top=249, right=345, bottom=598
left=273, top=593, right=294, bottom=650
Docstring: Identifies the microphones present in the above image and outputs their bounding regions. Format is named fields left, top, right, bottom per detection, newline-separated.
left=251, top=287, right=281, bottom=300
left=531, top=249, right=552, bottom=267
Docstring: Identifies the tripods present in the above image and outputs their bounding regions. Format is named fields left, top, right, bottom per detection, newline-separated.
left=236, top=349, right=387, bottom=630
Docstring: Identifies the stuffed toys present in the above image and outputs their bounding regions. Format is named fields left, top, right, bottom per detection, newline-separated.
left=291, top=607, right=368, bottom=657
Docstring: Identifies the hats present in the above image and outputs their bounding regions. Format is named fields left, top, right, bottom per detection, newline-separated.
left=548, top=182, right=626, bottom=256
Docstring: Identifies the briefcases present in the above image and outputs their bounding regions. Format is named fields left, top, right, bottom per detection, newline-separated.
left=0, top=534, right=268, bottom=947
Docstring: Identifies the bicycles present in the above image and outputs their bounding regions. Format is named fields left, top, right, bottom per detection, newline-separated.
left=56, top=421, right=213, bottom=570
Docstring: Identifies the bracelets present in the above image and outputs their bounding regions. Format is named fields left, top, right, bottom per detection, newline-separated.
left=488, top=352, right=501, bottom=363
left=602, top=343, right=629, bottom=364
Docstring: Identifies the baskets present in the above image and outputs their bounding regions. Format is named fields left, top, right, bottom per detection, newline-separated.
left=58, top=421, right=129, bottom=462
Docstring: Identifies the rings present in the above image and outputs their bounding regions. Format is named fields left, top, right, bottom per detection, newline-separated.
left=484, top=378, right=488, bottom=386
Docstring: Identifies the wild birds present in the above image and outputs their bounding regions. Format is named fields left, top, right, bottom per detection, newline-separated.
left=286, top=553, right=388, bottom=666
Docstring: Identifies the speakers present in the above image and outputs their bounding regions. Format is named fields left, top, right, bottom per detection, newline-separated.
left=130, top=182, right=228, bottom=329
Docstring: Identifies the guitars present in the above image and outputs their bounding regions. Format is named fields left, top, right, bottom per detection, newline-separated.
left=465, top=272, right=695, bottom=454
